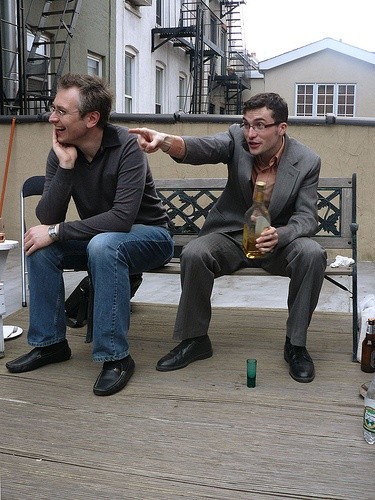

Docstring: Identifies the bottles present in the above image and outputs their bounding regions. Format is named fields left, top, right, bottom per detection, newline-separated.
left=362, top=318, right=374, bottom=373
left=243, top=181, right=272, bottom=259
left=363, top=376, right=374, bottom=445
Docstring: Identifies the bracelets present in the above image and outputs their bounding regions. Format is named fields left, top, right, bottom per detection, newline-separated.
left=48, top=224, right=58, bottom=242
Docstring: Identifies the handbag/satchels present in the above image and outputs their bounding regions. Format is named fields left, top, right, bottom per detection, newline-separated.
left=62, top=272, right=143, bottom=329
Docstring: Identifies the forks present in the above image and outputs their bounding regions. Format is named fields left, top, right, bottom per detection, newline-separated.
left=8, top=326, right=18, bottom=337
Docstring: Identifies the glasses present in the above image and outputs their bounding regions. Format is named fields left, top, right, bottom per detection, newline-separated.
left=239, top=121, right=279, bottom=130
left=48, top=105, right=84, bottom=117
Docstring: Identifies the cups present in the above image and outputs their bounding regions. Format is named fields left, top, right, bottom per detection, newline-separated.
left=247, top=359, right=257, bottom=387
left=0, top=218, right=5, bottom=243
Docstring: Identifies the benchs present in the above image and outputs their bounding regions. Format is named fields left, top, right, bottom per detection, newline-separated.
left=84, top=172, right=359, bottom=363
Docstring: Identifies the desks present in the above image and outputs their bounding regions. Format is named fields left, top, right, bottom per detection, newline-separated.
left=0, top=239, right=18, bottom=358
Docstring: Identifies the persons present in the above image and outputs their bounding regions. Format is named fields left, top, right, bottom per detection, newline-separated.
left=5, top=72, right=176, bottom=396
left=127, top=92, right=327, bottom=383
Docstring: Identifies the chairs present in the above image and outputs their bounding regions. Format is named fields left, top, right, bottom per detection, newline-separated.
left=20, top=176, right=78, bottom=307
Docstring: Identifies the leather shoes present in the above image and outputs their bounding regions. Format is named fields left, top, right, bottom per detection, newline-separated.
left=5, top=339, right=72, bottom=373
left=93, top=354, right=136, bottom=397
left=283, top=339, right=315, bottom=384
left=156, top=335, right=213, bottom=372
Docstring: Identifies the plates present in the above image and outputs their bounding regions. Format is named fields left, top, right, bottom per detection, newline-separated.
left=3, top=325, right=23, bottom=339
